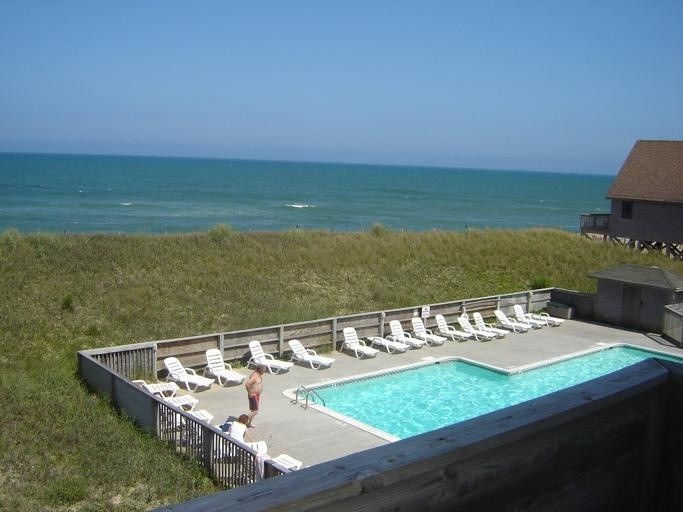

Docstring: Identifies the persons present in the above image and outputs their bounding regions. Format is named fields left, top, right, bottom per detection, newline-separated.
left=226, top=413, right=256, bottom=444
left=244, top=363, right=266, bottom=427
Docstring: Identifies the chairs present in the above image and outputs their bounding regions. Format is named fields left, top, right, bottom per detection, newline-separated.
left=134, top=379, right=180, bottom=398
left=286, top=339, right=335, bottom=369
left=523, top=312, right=563, bottom=327
left=456, top=316, right=496, bottom=342
left=366, top=336, right=410, bottom=354
left=513, top=305, right=547, bottom=331
left=233, top=452, right=303, bottom=484
left=411, top=318, right=447, bottom=347
left=493, top=309, right=532, bottom=335
left=181, top=422, right=234, bottom=450
left=200, top=440, right=269, bottom=463
left=163, top=357, right=216, bottom=393
left=156, top=410, right=216, bottom=441
left=385, top=320, right=426, bottom=348
left=472, top=311, right=510, bottom=341
left=156, top=393, right=199, bottom=411
left=433, top=314, right=473, bottom=343
left=202, top=349, right=245, bottom=387
left=246, top=340, right=295, bottom=374
left=339, top=327, right=380, bottom=359
